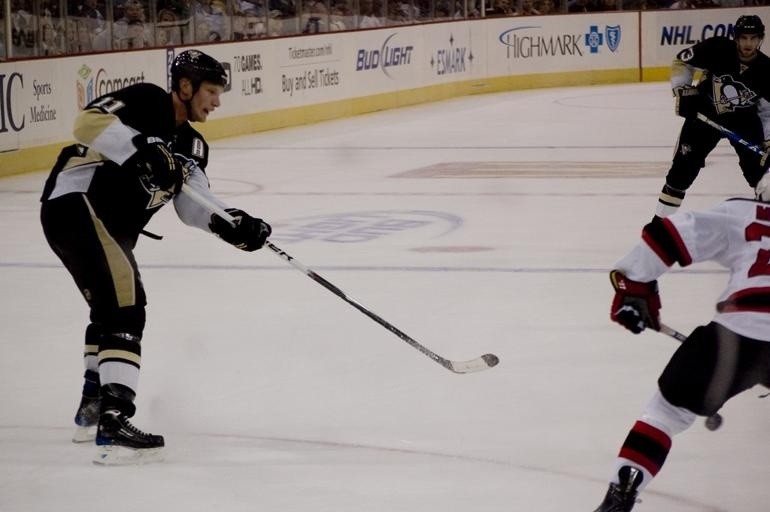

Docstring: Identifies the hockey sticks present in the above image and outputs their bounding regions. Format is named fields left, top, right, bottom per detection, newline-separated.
left=180, top=183, right=498, bottom=374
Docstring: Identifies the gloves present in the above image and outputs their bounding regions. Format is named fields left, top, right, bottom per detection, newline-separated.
left=610, top=269, right=661, bottom=334
left=672, top=84, right=701, bottom=118
left=759, top=139, right=770, bottom=169
left=208, top=209, right=271, bottom=251
left=131, top=132, right=184, bottom=196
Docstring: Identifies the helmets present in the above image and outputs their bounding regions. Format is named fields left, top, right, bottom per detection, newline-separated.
left=730, top=15, right=765, bottom=41
left=755, top=171, right=770, bottom=202
left=170, top=50, right=228, bottom=103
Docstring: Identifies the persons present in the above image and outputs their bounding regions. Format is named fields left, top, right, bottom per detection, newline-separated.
left=651, top=14, right=770, bottom=223
left=592, top=167, right=770, bottom=512
left=38, top=48, right=273, bottom=451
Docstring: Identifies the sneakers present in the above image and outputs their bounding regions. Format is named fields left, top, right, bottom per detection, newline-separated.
left=75, top=396, right=103, bottom=427
left=96, top=412, right=164, bottom=448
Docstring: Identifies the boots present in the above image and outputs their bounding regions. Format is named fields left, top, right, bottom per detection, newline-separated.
left=592, top=466, right=643, bottom=512
